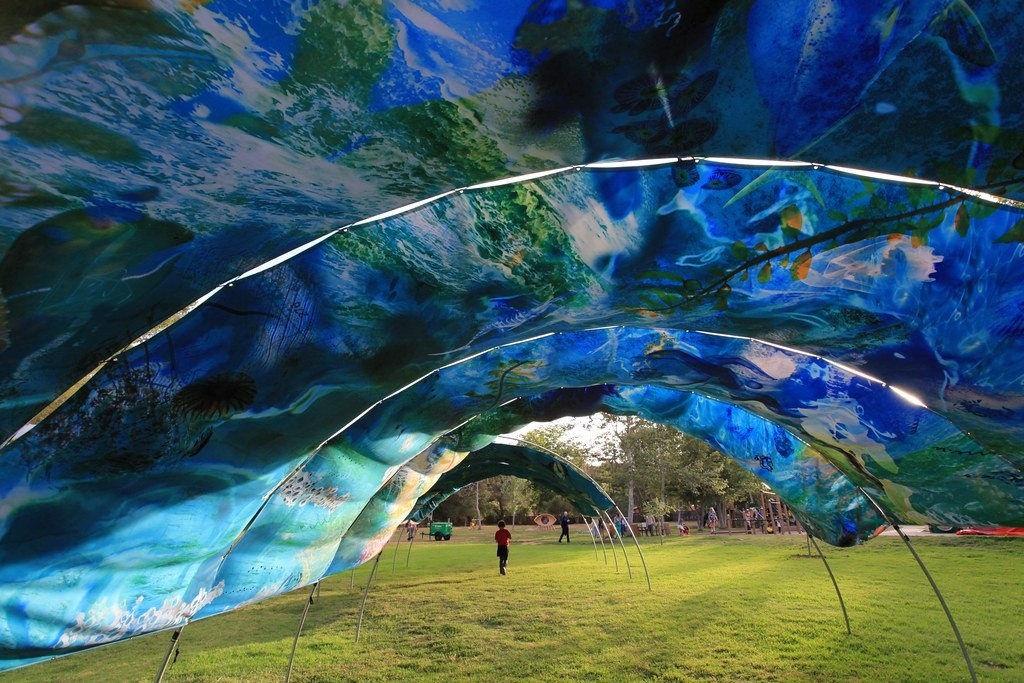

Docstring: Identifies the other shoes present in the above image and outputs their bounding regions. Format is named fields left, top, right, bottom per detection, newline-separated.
left=500, top=566, right=506, bottom=576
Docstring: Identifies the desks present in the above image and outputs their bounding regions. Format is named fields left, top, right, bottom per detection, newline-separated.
left=606, top=524, right=640, bottom=538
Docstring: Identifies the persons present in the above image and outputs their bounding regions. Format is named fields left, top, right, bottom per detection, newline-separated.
left=405, top=519, right=417, bottom=540
left=559, top=511, right=570, bottom=543
left=613, top=511, right=690, bottom=541
left=708, top=506, right=717, bottom=534
left=741, top=506, right=797, bottom=535
left=495, top=519, right=512, bottom=575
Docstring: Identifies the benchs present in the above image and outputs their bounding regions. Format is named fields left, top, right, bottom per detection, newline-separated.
left=637, top=526, right=667, bottom=536
